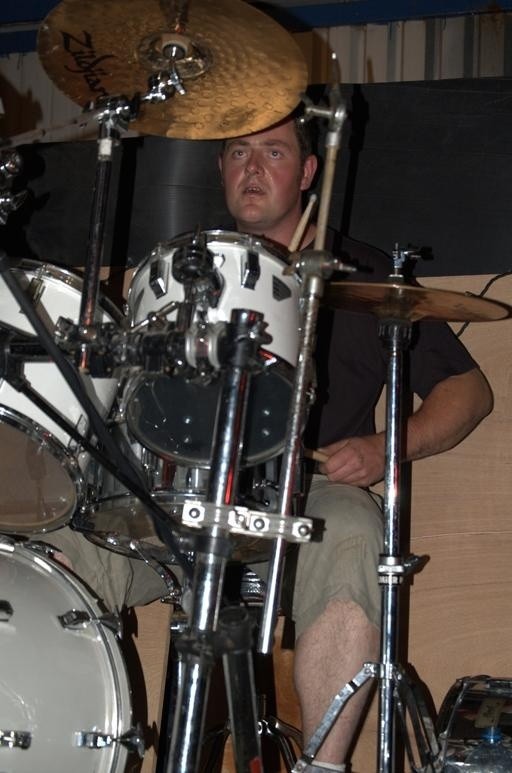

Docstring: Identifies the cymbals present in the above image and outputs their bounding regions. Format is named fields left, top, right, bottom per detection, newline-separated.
left=325, top=278, right=507, bottom=323
left=36, top=3, right=309, bottom=141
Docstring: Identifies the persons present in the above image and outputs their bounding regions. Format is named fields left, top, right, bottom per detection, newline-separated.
left=28, top=114, right=494, bottom=773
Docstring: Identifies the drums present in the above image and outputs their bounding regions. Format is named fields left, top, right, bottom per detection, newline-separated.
left=121, top=229, right=314, bottom=469
left=0, top=257, right=126, bottom=535
left=77, top=368, right=302, bottom=569
left=427, top=674, right=511, bottom=771
left=0, top=537, right=141, bottom=773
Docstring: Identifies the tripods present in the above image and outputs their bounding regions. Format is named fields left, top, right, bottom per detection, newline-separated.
left=285, top=342, right=442, bottom=773
